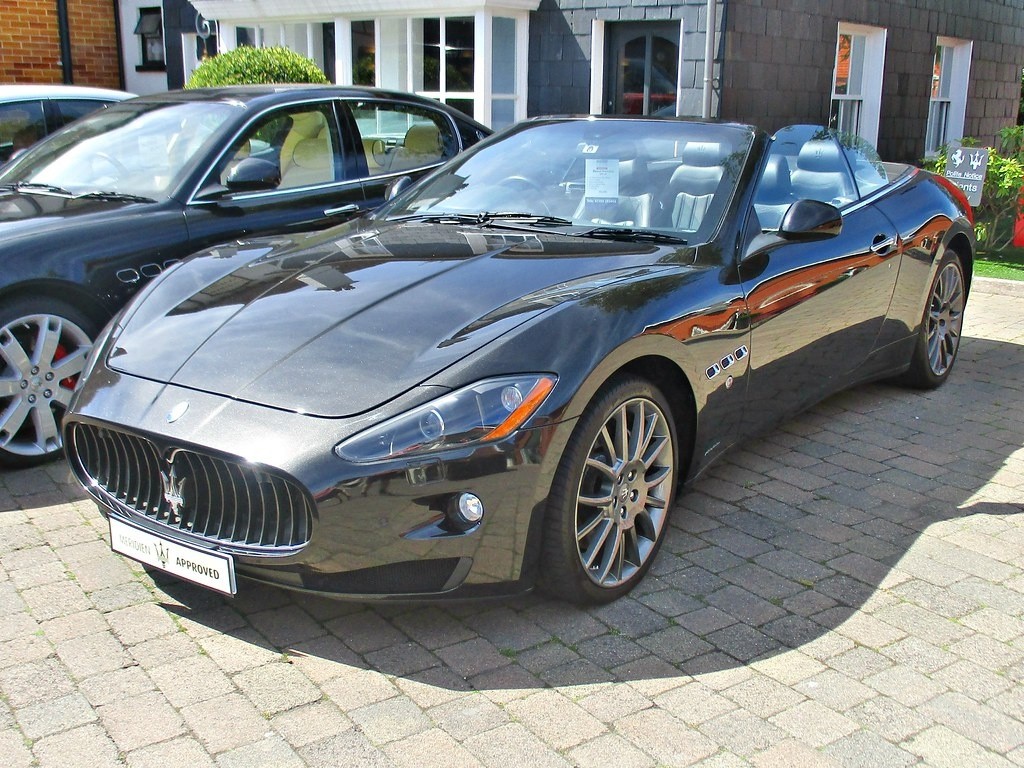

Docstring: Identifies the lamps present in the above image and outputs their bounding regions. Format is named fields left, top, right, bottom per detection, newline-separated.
left=194, top=12, right=212, bottom=68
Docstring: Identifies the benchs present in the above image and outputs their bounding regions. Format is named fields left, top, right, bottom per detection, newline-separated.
left=317, top=123, right=445, bottom=174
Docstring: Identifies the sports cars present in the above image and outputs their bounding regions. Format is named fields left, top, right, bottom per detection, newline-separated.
left=66, top=115, right=976, bottom=609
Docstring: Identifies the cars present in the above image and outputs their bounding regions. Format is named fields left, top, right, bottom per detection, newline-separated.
left=0, top=85, right=496, bottom=482
left=0, top=84, right=139, bottom=169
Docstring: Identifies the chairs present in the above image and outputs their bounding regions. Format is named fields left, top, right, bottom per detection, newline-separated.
left=572, top=154, right=658, bottom=229
left=755, top=153, right=798, bottom=230
left=791, top=140, right=856, bottom=201
left=201, top=136, right=250, bottom=188
left=277, top=137, right=333, bottom=189
left=664, top=137, right=732, bottom=233
